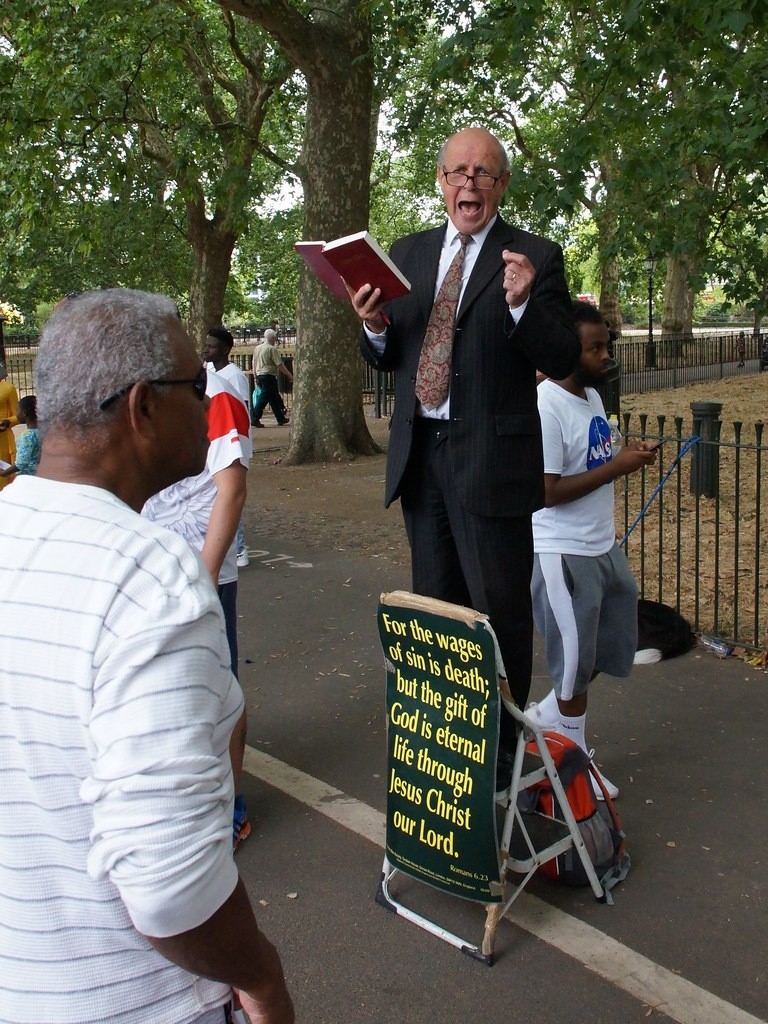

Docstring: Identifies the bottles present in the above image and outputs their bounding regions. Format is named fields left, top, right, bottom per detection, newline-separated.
left=606, top=414, right=623, bottom=459
left=697, top=636, right=732, bottom=659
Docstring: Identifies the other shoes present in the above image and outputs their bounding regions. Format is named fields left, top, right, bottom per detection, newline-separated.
left=277, top=417, right=290, bottom=426
left=251, top=421, right=264, bottom=428
left=496, top=749, right=515, bottom=794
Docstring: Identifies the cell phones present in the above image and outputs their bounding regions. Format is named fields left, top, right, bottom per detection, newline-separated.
left=645, top=435, right=672, bottom=452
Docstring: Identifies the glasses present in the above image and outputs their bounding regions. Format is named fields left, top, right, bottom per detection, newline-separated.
left=98, top=367, right=207, bottom=412
left=441, top=166, right=503, bottom=190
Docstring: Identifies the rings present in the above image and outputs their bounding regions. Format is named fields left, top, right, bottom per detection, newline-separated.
left=512, top=274, right=516, bottom=281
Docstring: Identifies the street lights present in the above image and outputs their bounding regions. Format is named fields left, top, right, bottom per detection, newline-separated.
left=642, top=251, right=660, bottom=367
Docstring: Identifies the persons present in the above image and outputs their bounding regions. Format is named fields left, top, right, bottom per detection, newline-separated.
left=340, top=128, right=583, bottom=791
left=605, top=319, right=616, bottom=358
left=0, top=396, right=41, bottom=477
left=0, top=285, right=296, bottom=1024
left=0, top=381, right=19, bottom=493
left=523, top=301, right=657, bottom=800
left=251, top=329, right=294, bottom=428
left=203, top=329, right=249, bottom=567
left=735, top=331, right=745, bottom=368
left=139, top=370, right=251, bottom=854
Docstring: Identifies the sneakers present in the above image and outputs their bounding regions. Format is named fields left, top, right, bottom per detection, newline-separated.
left=231, top=793, right=253, bottom=855
left=587, top=748, right=619, bottom=801
left=521, top=702, right=563, bottom=745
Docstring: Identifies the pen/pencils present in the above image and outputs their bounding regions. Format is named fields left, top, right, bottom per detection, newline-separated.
left=380, top=311, right=391, bottom=326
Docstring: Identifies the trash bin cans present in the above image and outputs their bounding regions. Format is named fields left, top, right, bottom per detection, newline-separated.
left=277, top=357, right=294, bottom=394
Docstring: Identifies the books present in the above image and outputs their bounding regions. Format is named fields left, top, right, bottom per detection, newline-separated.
left=292, top=230, right=411, bottom=306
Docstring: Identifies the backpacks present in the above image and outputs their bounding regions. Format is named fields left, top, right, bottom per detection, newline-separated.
left=516, top=731, right=633, bottom=905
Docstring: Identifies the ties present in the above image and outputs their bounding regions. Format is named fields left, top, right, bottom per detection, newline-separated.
left=414, top=232, right=473, bottom=412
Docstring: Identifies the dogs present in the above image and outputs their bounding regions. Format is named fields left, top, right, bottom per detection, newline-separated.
left=633, top=600, right=694, bottom=665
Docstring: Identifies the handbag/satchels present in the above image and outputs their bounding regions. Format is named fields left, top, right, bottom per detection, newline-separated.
left=251, top=384, right=263, bottom=408
